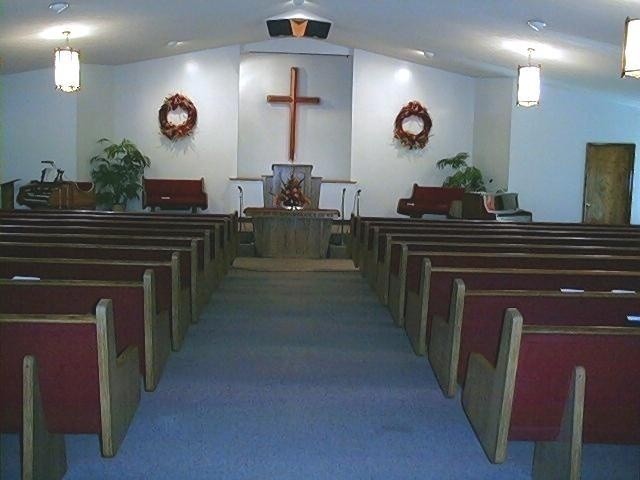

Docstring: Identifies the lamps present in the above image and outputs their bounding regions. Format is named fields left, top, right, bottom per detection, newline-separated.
left=621, top=15, right=640, bottom=81
left=265, top=0, right=333, bottom=40
left=54, top=30, right=81, bottom=94
left=518, top=47, right=541, bottom=109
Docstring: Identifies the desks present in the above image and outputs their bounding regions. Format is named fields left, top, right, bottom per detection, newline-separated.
left=243, top=208, right=340, bottom=259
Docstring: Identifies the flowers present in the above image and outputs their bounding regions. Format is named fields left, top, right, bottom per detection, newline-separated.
left=159, top=95, right=198, bottom=139
left=394, top=101, right=431, bottom=150
left=272, top=166, right=312, bottom=211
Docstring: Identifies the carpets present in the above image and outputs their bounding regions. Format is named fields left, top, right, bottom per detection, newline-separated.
left=233, top=257, right=356, bottom=273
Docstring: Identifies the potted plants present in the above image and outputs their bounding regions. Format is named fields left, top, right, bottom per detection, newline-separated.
left=89, top=137, right=152, bottom=211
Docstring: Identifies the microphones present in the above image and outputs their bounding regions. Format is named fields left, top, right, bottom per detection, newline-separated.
left=357, top=189, right=361, bottom=194
left=475, top=179, right=493, bottom=192
left=238, top=185, right=243, bottom=191
left=41, top=160, right=55, bottom=164
left=342, top=188, right=346, bottom=193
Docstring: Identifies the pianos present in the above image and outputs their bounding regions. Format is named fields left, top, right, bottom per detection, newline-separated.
left=397, top=183, right=532, bottom=221
left=17, top=180, right=95, bottom=210
left=141, top=176, right=208, bottom=212
